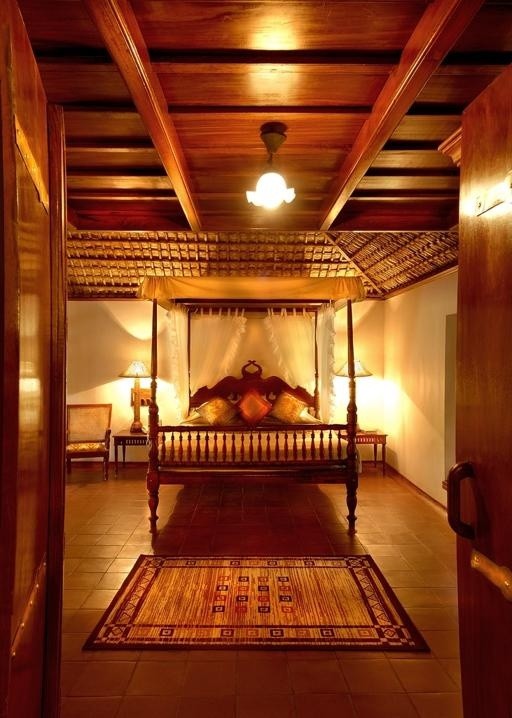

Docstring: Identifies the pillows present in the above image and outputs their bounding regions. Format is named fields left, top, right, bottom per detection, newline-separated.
left=235, top=389, right=274, bottom=425
left=270, top=391, right=308, bottom=424
left=180, top=401, right=323, bottom=426
left=195, top=396, right=238, bottom=426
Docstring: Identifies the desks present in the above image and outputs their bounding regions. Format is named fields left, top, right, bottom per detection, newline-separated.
left=335, top=429, right=388, bottom=477
left=112, top=430, right=163, bottom=480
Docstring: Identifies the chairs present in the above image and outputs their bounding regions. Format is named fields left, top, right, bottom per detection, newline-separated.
left=66, top=404, right=112, bottom=480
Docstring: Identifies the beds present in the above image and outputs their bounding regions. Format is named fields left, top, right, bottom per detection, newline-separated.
left=136, top=275, right=366, bottom=534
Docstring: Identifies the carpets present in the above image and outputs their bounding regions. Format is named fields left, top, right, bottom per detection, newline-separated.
left=83, top=555, right=430, bottom=660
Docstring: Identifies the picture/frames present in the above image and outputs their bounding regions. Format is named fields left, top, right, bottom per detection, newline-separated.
left=131, top=388, right=155, bottom=407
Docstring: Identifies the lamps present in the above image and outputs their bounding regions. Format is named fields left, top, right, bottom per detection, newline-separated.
left=335, top=360, right=373, bottom=431
left=119, top=360, right=151, bottom=432
left=246, top=130, right=296, bottom=210
